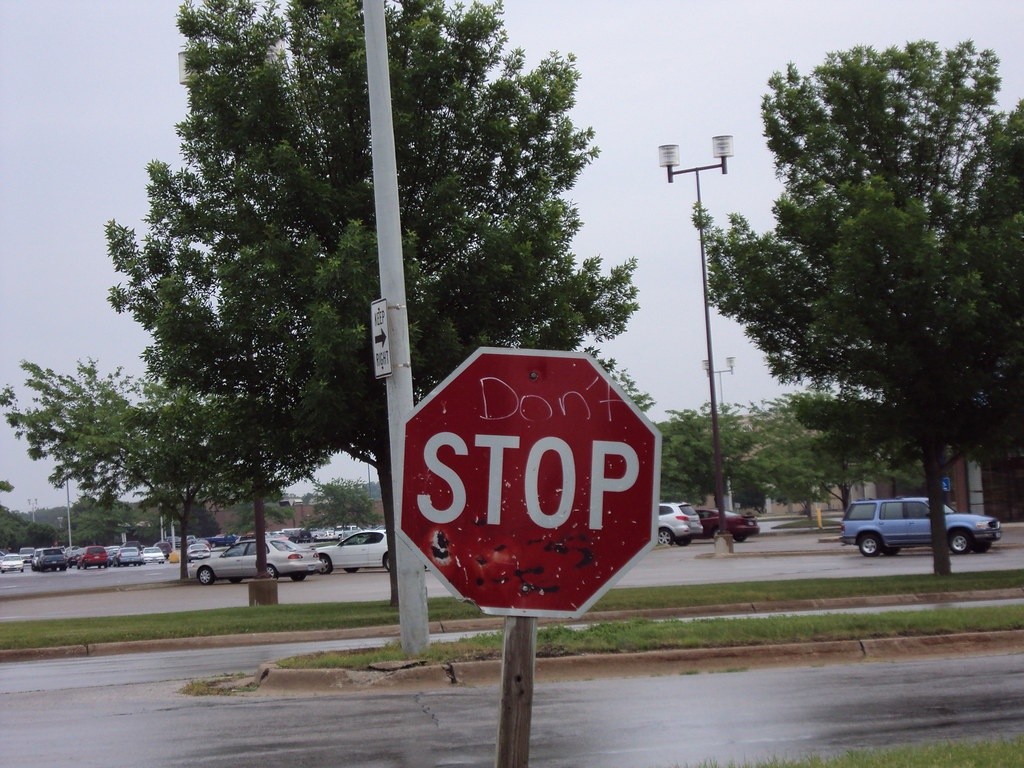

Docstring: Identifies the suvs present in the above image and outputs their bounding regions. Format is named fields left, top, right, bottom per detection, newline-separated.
left=657, top=502, right=704, bottom=548
left=837, top=496, right=1003, bottom=558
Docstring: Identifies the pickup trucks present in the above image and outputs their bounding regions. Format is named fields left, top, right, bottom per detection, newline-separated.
left=204, top=533, right=239, bottom=548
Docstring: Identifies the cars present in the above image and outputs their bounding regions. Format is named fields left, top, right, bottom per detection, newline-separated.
left=0, top=533, right=208, bottom=573
left=187, top=544, right=211, bottom=564
left=187, top=538, right=323, bottom=586
left=230, top=524, right=386, bottom=544
left=692, top=509, right=761, bottom=543
left=315, top=529, right=389, bottom=576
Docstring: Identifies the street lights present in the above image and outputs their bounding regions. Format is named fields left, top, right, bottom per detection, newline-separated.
left=56, top=517, right=64, bottom=529
left=656, top=134, right=735, bottom=537
left=701, top=356, right=737, bottom=416
left=28, top=498, right=38, bottom=522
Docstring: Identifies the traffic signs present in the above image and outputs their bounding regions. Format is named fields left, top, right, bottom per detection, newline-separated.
left=369, top=297, right=394, bottom=380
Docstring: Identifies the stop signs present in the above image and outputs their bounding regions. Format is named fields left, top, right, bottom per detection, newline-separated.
left=393, top=345, right=662, bottom=620
